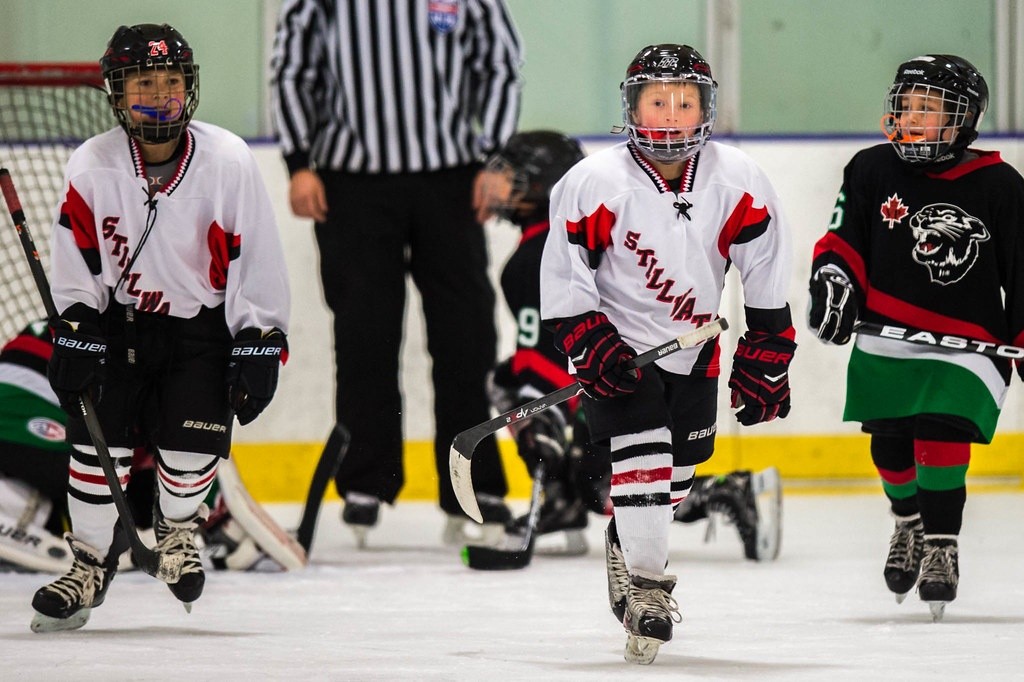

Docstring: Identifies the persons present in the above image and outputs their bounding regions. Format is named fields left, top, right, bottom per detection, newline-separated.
left=806, top=54, right=1024, bottom=624
left=1, top=0, right=798, bottom=665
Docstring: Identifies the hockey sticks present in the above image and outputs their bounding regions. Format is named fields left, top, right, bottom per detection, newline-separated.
left=449, top=316, right=730, bottom=525
left=0, top=167, right=185, bottom=585
left=852, top=317, right=1024, bottom=363
left=459, top=462, right=546, bottom=571
left=245, top=425, right=352, bottom=573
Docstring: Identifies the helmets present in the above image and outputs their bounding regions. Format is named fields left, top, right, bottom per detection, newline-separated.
left=98, top=24, right=200, bottom=143
left=484, top=132, right=585, bottom=227
left=881, top=54, right=989, bottom=166
left=620, top=43, right=719, bottom=165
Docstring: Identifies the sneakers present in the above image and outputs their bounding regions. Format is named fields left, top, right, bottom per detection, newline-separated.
left=152, top=493, right=205, bottom=614
left=30, top=530, right=120, bottom=633
left=622, top=568, right=677, bottom=665
left=442, top=490, right=513, bottom=555
left=608, top=516, right=630, bottom=626
left=708, top=464, right=781, bottom=562
left=508, top=479, right=590, bottom=557
left=919, top=538, right=960, bottom=622
left=883, top=516, right=924, bottom=603
left=341, top=491, right=380, bottom=549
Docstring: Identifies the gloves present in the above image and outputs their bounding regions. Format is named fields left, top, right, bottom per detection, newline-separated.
left=553, top=311, right=642, bottom=402
left=47, top=319, right=110, bottom=417
left=728, top=329, right=798, bottom=426
left=227, top=327, right=288, bottom=425
left=808, top=268, right=861, bottom=347
